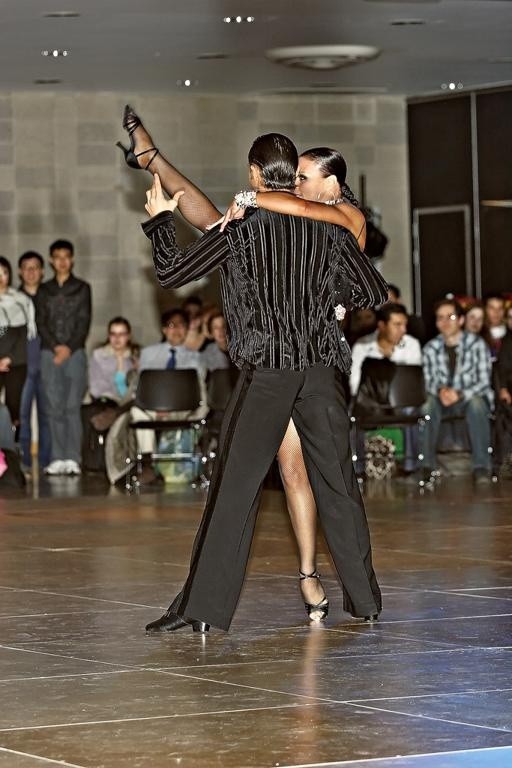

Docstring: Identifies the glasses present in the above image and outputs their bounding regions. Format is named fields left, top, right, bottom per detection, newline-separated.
left=1, top=270, right=8, bottom=276
left=109, top=331, right=128, bottom=338
left=164, top=321, right=188, bottom=330
left=22, top=265, right=40, bottom=273
left=437, top=314, right=457, bottom=321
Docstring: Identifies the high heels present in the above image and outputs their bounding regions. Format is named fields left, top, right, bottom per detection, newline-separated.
left=115, top=104, right=159, bottom=171
left=298, top=570, right=329, bottom=621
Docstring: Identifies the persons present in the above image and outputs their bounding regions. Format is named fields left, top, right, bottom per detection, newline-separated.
left=140, top=132, right=388, bottom=633
left=350, top=308, right=376, bottom=328
left=35, top=240, right=91, bottom=474
left=0, top=256, right=39, bottom=489
left=199, top=316, right=236, bottom=372
left=137, top=308, right=208, bottom=489
left=15, top=250, right=49, bottom=480
left=377, top=283, right=428, bottom=349
left=506, top=306, right=512, bottom=329
left=418, top=299, right=494, bottom=494
left=117, top=105, right=366, bottom=620
left=1, top=388, right=27, bottom=491
left=349, top=303, right=440, bottom=495
left=201, top=304, right=220, bottom=339
left=83, top=315, right=156, bottom=484
left=483, top=298, right=512, bottom=481
left=182, top=294, right=209, bottom=353
left=461, top=304, right=484, bottom=332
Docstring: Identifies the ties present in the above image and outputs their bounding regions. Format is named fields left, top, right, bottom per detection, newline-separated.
left=166, top=349, right=176, bottom=368
left=115, top=352, right=127, bottom=396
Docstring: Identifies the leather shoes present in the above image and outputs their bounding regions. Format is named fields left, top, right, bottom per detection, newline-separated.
left=345, top=609, right=378, bottom=624
left=146, top=610, right=210, bottom=635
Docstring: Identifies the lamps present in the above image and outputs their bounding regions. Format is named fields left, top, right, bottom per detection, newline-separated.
left=265, top=43, right=382, bottom=70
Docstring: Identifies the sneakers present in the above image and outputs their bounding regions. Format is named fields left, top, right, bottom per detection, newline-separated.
left=43, top=459, right=62, bottom=477
left=470, top=469, right=495, bottom=488
left=61, top=459, right=81, bottom=476
left=418, top=469, right=437, bottom=490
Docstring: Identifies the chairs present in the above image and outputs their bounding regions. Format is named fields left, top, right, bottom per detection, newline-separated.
left=439, top=356, right=496, bottom=479
left=125, top=367, right=210, bottom=495
left=204, top=366, right=277, bottom=491
left=351, top=364, right=431, bottom=495
